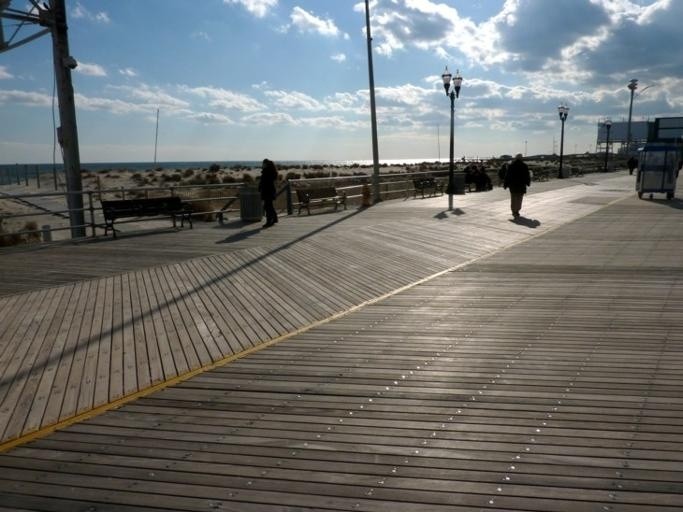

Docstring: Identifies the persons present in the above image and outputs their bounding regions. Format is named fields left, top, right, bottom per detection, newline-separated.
left=626, top=156, right=634, bottom=174
left=503, top=153, right=531, bottom=218
left=463, top=164, right=493, bottom=192
left=497, top=163, right=506, bottom=187
left=256, top=158, right=278, bottom=227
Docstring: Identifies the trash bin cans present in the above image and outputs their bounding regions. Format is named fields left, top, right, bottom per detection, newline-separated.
left=453, top=173, right=466, bottom=194
left=240, top=192, right=262, bottom=222
left=562, top=168, right=569, bottom=177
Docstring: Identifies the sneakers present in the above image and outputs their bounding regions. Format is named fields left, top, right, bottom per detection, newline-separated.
left=262, top=219, right=278, bottom=228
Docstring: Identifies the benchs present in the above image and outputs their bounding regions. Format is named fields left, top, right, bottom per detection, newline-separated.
left=413, top=177, right=444, bottom=198
left=295, top=187, right=348, bottom=215
left=100, top=196, right=194, bottom=240
